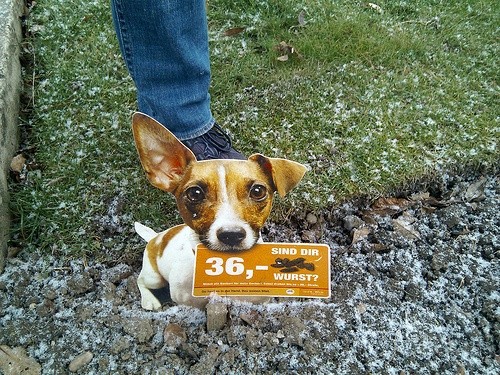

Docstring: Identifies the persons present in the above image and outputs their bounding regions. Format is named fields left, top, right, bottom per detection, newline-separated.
left=108, top=0, right=243, bottom=162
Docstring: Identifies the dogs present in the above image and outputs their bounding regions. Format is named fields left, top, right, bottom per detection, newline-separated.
left=131, top=111, right=306, bottom=311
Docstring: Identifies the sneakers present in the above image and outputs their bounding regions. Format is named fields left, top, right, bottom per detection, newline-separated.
left=184, top=126, right=247, bottom=160
